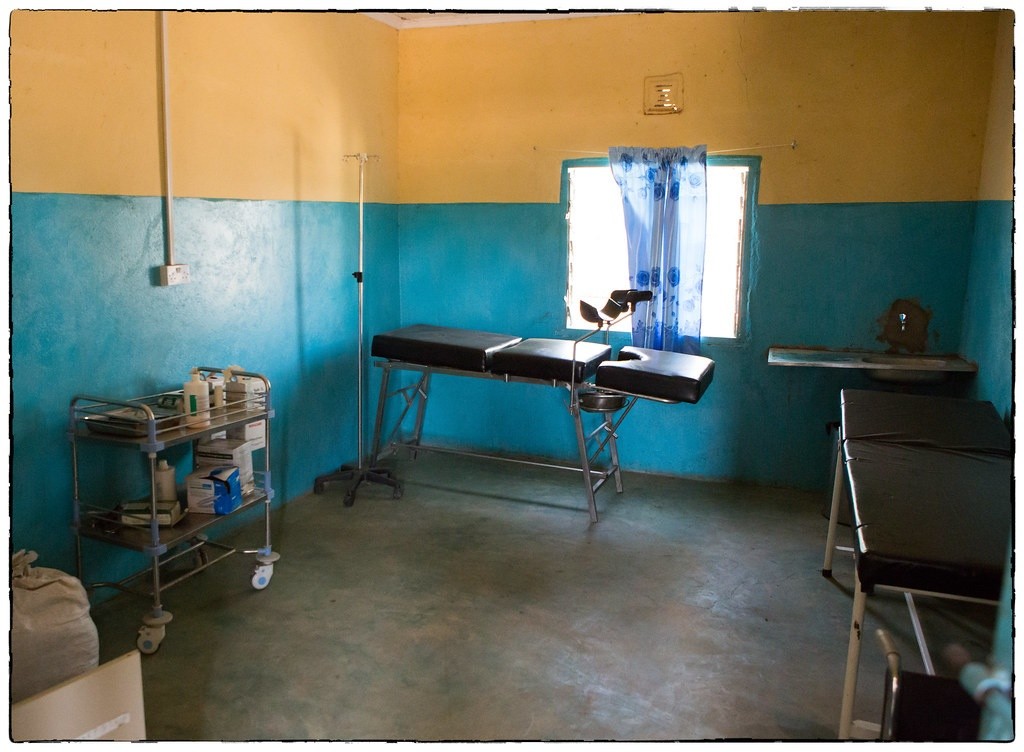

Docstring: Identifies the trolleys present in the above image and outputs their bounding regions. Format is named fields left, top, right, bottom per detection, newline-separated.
left=67, top=366, right=282, bottom=656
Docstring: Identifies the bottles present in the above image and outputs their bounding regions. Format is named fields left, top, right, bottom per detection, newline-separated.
left=156, top=460, right=177, bottom=502
left=184, top=373, right=210, bottom=427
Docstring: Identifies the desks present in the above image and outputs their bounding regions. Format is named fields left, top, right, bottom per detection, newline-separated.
left=767, top=346, right=978, bottom=374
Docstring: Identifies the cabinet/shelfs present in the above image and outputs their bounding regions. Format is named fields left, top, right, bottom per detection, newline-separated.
left=67, top=366, right=280, bottom=654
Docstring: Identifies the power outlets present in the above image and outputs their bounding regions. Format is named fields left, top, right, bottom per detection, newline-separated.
left=160, top=264, right=190, bottom=284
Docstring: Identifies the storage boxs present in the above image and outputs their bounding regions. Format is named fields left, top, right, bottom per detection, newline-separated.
left=226, top=378, right=269, bottom=451
left=120, top=500, right=180, bottom=526
left=196, top=436, right=255, bottom=497
left=186, top=465, right=244, bottom=514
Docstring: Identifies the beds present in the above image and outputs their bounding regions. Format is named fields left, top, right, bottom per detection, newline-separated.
left=820, top=388, right=1015, bottom=742
left=369, top=319, right=717, bottom=522
